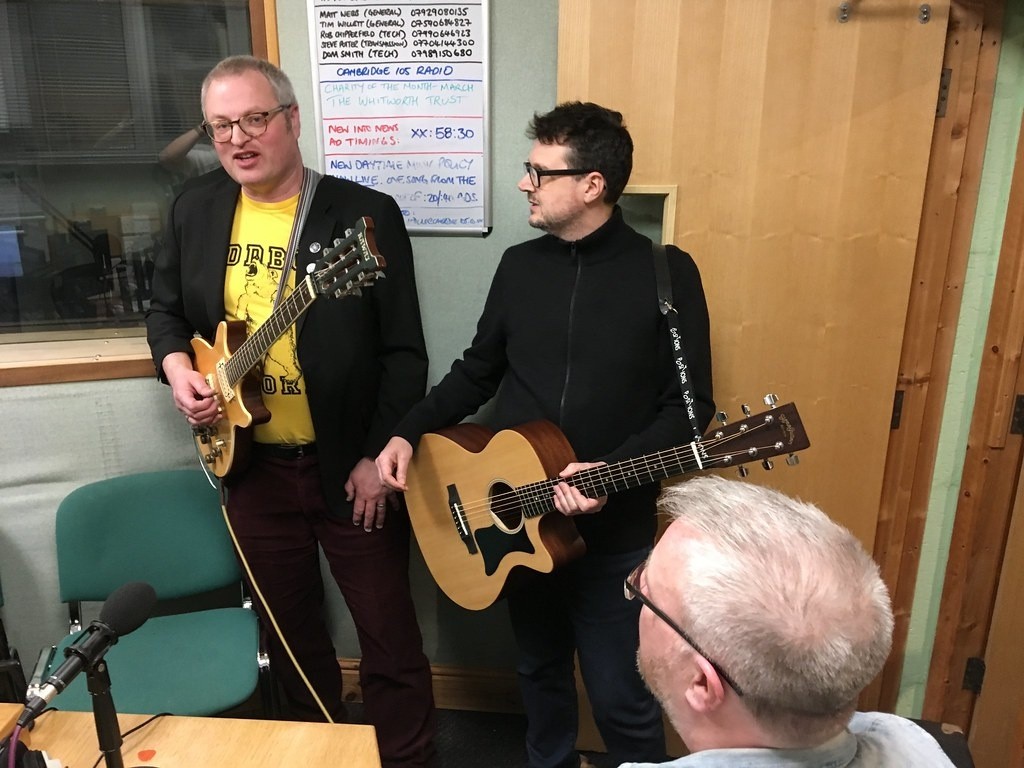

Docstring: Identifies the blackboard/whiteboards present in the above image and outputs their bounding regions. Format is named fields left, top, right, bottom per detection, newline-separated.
left=307, top=0, right=493, bottom=236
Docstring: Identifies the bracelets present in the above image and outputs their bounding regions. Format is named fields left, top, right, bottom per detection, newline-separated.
left=194, top=126, right=204, bottom=138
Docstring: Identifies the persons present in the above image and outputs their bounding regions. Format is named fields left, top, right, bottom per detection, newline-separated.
left=623, top=477, right=957, bottom=768
left=375, top=101, right=716, bottom=768
left=159, top=120, right=222, bottom=177
left=144, top=54, right=436, bottom=768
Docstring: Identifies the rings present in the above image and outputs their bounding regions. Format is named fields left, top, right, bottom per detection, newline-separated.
left=377, top=504, right=386, bottom=508
left=185, top=414, right=189, bottom=423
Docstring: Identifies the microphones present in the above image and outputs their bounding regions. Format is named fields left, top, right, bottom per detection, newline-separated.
left=17, top=582, right=156, bottom=727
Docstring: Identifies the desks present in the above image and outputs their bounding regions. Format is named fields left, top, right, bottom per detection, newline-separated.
left=0, top=704, right=381, bottom=768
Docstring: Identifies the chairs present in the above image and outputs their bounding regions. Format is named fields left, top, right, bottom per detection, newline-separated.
left=25, top=469, right=274, bottom=715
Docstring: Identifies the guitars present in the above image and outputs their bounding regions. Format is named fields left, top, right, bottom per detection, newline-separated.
left=401, top=393, right=812, bottom=613
left=187, top=215, right=387, bottom=480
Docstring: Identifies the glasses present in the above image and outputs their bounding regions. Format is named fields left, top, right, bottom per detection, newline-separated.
left=523, top=161, right=606, bottom=190
left=201, top=104, right=291, bottom=143
left=623, top=518, right=744, bottom=698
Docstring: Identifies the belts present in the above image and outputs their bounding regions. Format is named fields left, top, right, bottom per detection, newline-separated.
left=252, top=440, right=316, bottom=460
